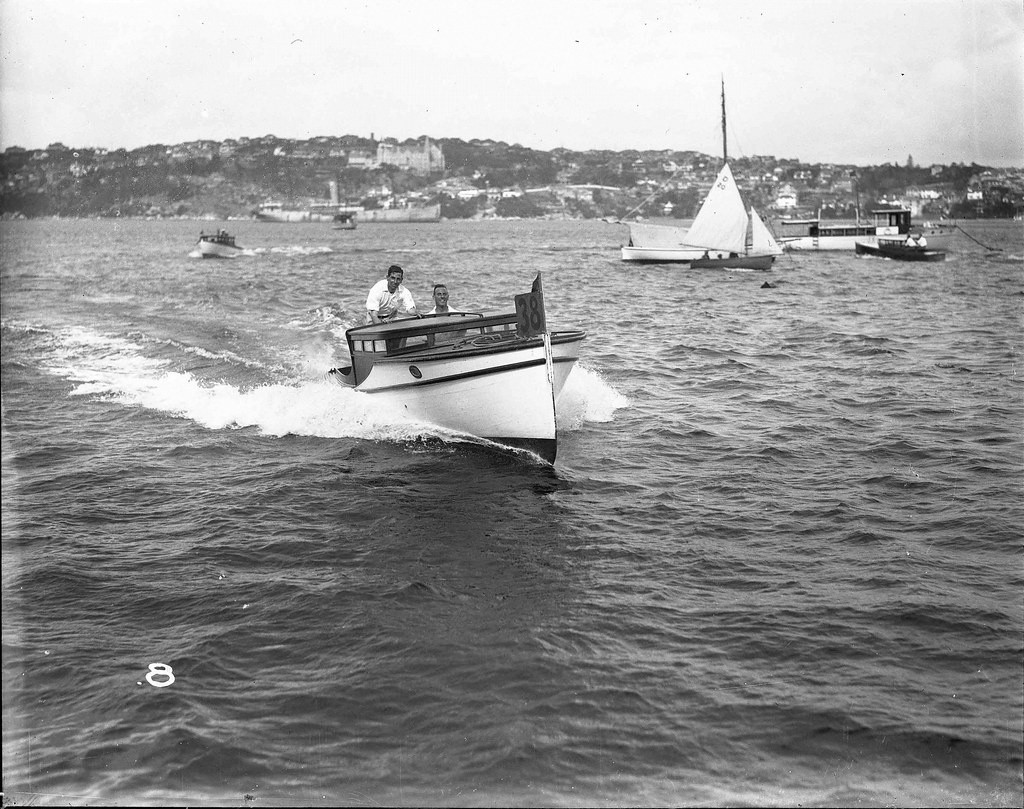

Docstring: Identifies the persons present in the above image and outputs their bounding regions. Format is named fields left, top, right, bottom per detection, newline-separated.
left=430, top=284, right=458, bottom=313
left=906, top=235, right=916, bottom=247
left=702, top=251, right=709, bottom=259
left=366, top=265, right=418, bottom=324
left=917, top=233, right=927, bottom=246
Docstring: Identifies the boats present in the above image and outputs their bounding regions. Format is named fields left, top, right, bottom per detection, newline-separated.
left=855, top=237, right=947, bottom=262
left=197, top=228, right=245, bottom=259
left=909, top=220, right=958, bottom=236
left=324, top=270, right=588, bottom=468
left=330, top=197, right=361, bottom=231
left=746, top=169, right=921, bottom=252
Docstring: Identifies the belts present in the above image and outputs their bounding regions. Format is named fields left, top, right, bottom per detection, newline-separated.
left=378, top=315, right=389, bottom=319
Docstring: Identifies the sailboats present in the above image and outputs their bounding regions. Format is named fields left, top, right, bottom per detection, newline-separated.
left=622, top=72, right=787, bottom=269
